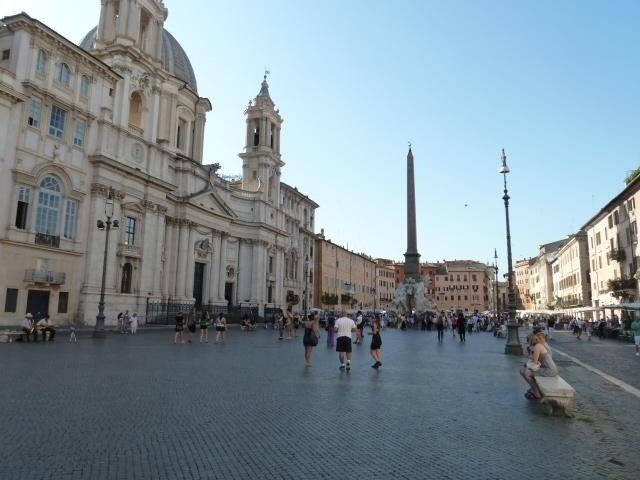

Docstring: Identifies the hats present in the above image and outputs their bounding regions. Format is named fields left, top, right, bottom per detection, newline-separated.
left=24, top=312, right=34, bottom=318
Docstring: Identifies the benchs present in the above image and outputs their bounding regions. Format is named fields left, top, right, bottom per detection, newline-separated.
left=531, top=368, right=575, bottom=418
left=3, top=329, right=54, bottom=343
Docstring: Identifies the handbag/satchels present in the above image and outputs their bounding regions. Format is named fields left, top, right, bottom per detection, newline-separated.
left=310, top=333, right=319, bottom=347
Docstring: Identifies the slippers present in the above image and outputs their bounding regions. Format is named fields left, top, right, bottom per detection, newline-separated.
left=524, top=389, right=542, bottom=400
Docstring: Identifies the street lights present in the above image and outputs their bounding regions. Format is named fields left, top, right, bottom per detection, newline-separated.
left=371, top=287, right=377, bottom=314
left=303, top=254, right=310, bottom=318
left=492, top=249, right=498, bottom=327
left=91, top=184, right=120, bottom=335
left=498, top=148, right=523, bottom=354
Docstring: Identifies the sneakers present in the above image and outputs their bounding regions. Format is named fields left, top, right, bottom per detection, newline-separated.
left=277, top=333, right=299, bottom=343
left=436, top=341, right=443, bottom=345
left=353, top=340, right=362, bottom=346
left=173, top=339, right=227, bottom=347
left=635, top=352, right=640, bottom=358
left=458, top=339, right=467, bottom=344
left=304, top=359, right=388, bottom=372
